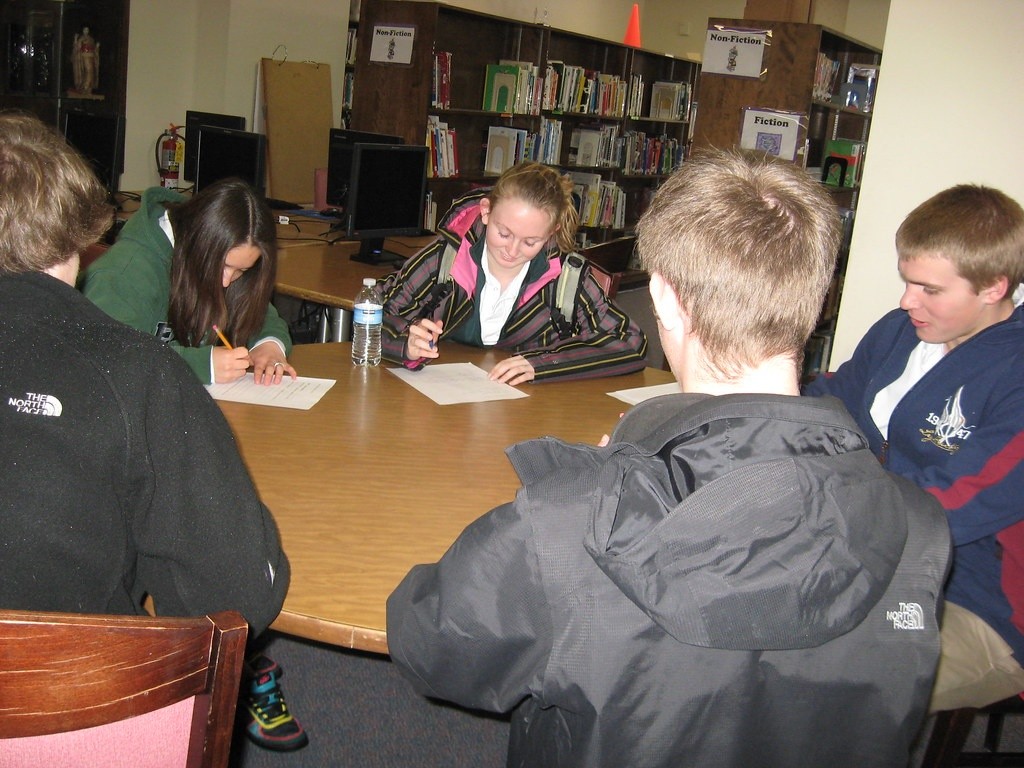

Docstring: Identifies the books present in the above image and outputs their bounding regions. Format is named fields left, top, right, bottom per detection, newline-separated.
left=458, top=173, right=627, bottom=231
left=819, top=137, right=864, bottom=186
left=484, top=116, right=688, bottom=174
left=422, top=185, right=438, bottom=232
left=481, top=59, right=693, bottom=123
left=810, top=51, right=841, bottom=102
left=423, top=116, right=461, bottom=180
left=432, top=51, right=452, bottom=110
left=800, top=201, right=852, bottom=383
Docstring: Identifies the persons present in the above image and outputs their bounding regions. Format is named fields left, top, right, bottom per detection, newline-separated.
left=358, top=160, right=648, bottom=386
left=69, top=25, right=100, bottom=93
left=387, top=142, right=951, bottom=768
left=1, top=113, right=291, bottom=634
left=72, top=174, right=309, bottom=752
left=801, top=185, right=1022, bottom=716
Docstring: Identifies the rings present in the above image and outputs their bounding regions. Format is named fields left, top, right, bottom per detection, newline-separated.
left=273, top=362, right=285, bottom=371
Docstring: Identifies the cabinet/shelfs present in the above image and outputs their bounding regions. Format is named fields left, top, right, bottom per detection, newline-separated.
left=692, top=15, right=883, bottom=394
left=348, top=0, right=702, bottom=270
left=0, top=0, right=131, bottom=191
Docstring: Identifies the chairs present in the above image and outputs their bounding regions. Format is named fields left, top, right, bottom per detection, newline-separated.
left=0, top=598, right=249, bottom=768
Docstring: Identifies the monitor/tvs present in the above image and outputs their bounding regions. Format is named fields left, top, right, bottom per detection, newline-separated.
left=183, top=109, right=267, bottom=197
left=60, top=105, right=125, bottom=210
left=322, top=128, right=430, bottom=268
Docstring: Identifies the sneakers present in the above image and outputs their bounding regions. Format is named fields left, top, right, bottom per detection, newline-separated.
left=236, top=649, right=307, bottom=753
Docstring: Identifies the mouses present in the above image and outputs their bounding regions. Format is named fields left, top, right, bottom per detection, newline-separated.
left=322, top=207, right=340, bottom=215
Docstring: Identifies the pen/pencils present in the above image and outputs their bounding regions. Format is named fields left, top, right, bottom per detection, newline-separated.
left=212, top=325, right=233, bottom=350
left=428, top=316, right=433, bottom=350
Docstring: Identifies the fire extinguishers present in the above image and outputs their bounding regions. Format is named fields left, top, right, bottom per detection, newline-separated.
left=155, top=123, right=186, bottom=190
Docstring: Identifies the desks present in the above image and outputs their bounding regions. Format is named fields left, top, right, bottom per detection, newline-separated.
left=276, top=230, right=440, bottom=341
left=104, top=186, right=357, bottom=247
left=213, top=338, right=674, bottom=656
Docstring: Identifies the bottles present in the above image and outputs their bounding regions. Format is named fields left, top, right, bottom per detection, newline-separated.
left=352, top=278, right=384, bottom=368
